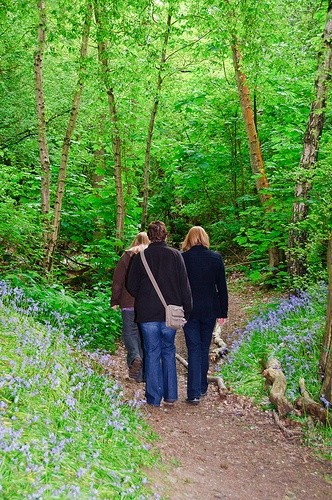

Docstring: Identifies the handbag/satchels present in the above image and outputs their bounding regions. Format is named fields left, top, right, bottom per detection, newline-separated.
left=165, top=304, right=187, bottom=330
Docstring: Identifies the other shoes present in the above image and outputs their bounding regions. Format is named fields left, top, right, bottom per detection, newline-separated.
left=201, top=393, right=208, bottom=397
left=162, top=399, right=174, bottom=408
left=129, top=357, right=142, bottom=379
left=140, top=400, right=159, bottom=410
left=186, top=397, right=200, bottom=405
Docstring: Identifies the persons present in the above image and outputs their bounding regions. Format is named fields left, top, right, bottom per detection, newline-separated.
left=110, top=219, right=229, bottom=409
left=124, top=219, right=194, bottom=409
